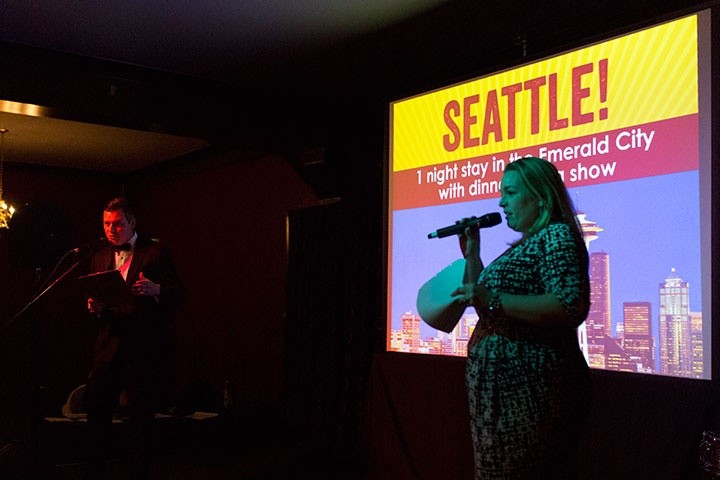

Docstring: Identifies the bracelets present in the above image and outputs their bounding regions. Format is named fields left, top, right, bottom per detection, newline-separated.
left=485, top=291, right=505, bottom=317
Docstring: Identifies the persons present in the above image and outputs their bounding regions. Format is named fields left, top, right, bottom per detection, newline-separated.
left=450, top=156, right=591, bottom=480
left=80, top=197, right=191, bottom=425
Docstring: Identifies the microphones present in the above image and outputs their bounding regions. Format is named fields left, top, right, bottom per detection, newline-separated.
left=428, top=212, right=502, bottom=238
left=74, top=236, right=106, bottom=253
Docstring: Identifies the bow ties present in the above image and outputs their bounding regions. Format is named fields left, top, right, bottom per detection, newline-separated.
left=115, top=243, right=132, bottom=251
